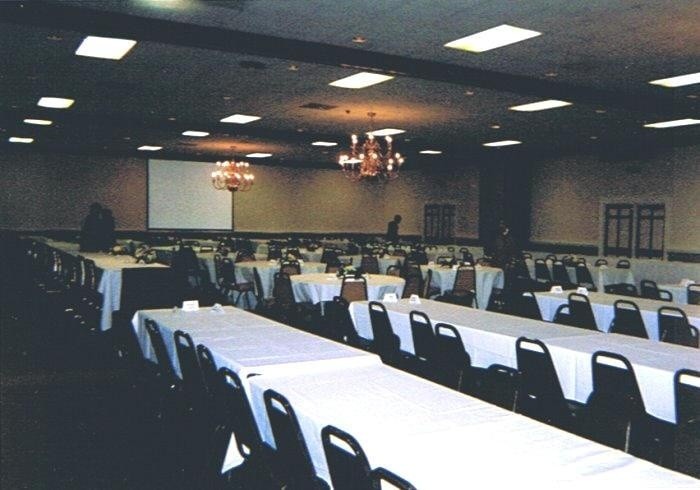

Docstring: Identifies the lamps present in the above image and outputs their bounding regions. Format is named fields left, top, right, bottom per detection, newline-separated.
left=210, top=144, right=254, bottom=192
left=336, top=113, right=408, bottom=186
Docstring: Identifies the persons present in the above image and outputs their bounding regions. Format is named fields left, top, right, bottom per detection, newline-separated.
left=386, top=213, right=401, bottom=238
left=102, top=208, right=115, bottom=250
left=80, top=202, right=102, bottom=250
left=484, top=219, right=525, bottom=268
